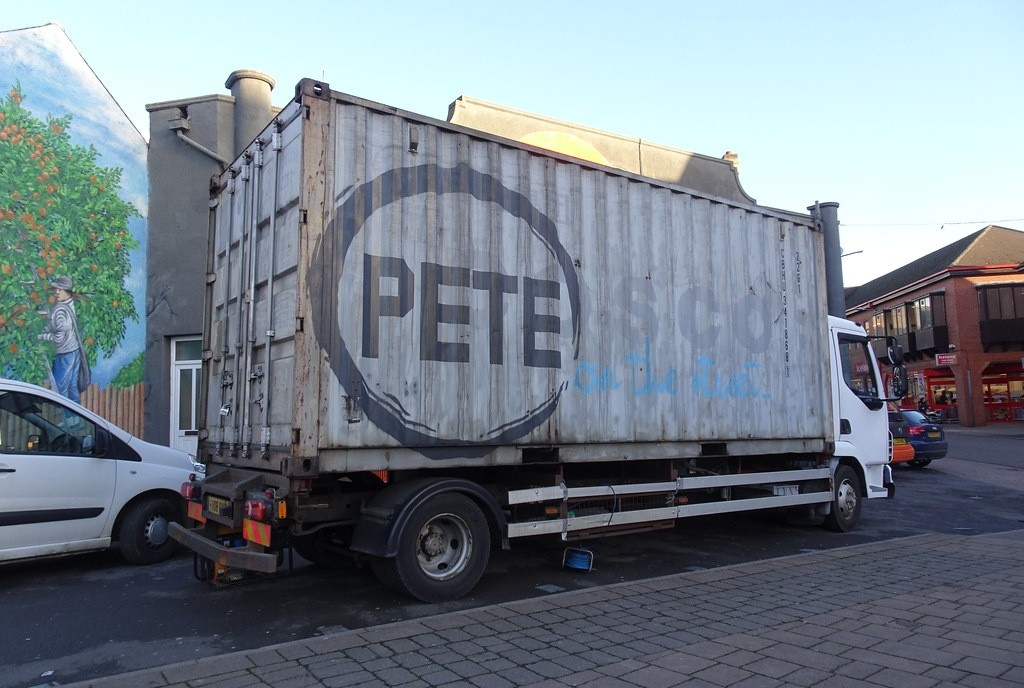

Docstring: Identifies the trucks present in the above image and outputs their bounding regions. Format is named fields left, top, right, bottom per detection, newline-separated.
left=162, top=77, right=908, bottom=605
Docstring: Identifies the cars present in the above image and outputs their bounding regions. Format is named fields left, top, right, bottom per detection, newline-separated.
left=888, top=408, right=949, bottom=468
left=0, top=375, right=209, bottom=571
